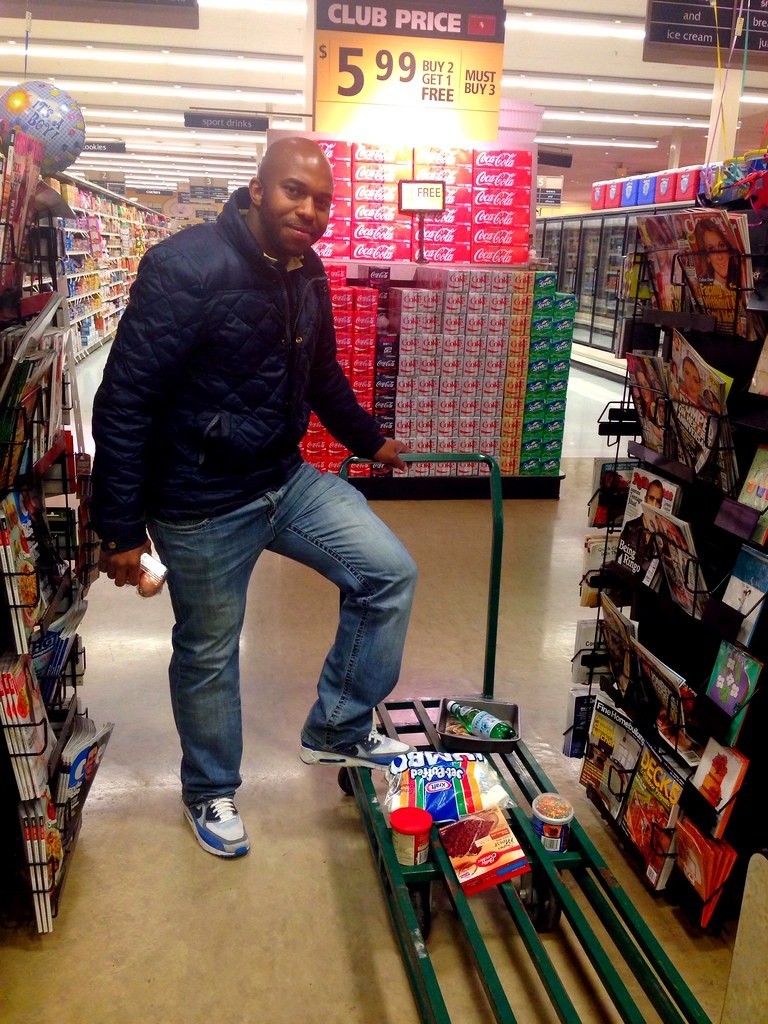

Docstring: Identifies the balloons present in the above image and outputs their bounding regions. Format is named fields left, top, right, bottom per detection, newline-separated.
left=0, top=81, right=85, bottom=177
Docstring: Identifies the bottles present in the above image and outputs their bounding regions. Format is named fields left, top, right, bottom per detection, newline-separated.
left=447, top=700, right=516, bottom=739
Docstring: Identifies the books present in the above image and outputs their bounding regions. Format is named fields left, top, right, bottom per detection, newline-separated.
left=0, top=129, right=115, bottom=935
left=563, top=207, right=768, bottom=927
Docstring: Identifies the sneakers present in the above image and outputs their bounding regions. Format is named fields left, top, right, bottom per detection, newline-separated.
left=182, top=796, right=249, bottom=857
left=299, top=730, right=417, bottom=772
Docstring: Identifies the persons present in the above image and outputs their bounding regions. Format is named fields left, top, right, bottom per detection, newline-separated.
left=632, top=362, right=662, bottom=441
left=679, top=356, right=700, bottom=404
left=693, top=216, right=740, bottom=293
left=621, top=479, right=663, bottom=572
left=674, top=219, right=687, bottom=241
left=643, top=217, right=673, bottom=282
left=87, top=139, right=418, bottom=860
left=668, top=360, right=680, bottom=398
left=655, top=216, right=676, bottom=249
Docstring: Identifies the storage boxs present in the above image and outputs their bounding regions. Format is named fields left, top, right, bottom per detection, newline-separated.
left=296, top=139, right=577, bottom=479
left=441, top=805, right=530, bottom=897
left=589, top=162, right=721, bottom=211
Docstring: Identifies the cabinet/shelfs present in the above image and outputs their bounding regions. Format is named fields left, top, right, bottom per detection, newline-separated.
left=533, top=214, right=768, bottom=936
left=0, top=124, right=170, bottom=938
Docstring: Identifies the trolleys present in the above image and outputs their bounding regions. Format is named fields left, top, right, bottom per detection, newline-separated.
left=337, top=452, right=712, bottom=1024
